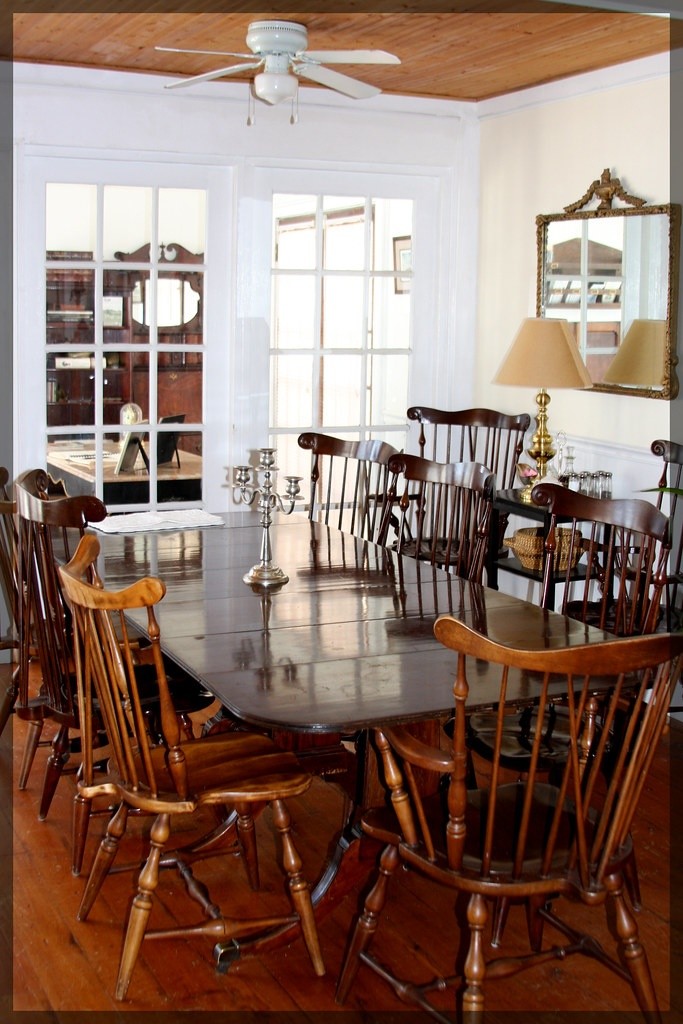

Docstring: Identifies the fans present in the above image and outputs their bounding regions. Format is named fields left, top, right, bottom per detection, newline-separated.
left=154, top=21, right=402, bottom=125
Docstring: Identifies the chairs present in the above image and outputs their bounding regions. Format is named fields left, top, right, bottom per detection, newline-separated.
left=0, top=407, right=683, bottom=1024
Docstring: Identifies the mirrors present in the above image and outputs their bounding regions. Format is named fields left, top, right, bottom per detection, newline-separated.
left=536, top=167, right=680, bottom=400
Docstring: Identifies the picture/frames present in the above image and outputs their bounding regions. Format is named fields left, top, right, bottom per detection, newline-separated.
left=115, top=420, right=150, bottom=475
left=158, top=415, right=187, bottom=469
left=393, top=235, right=411, bottom=294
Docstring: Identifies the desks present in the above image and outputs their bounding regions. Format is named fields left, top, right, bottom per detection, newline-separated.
left=40, top=510, right=655, bottom=971
left=47, top=440, right=202, bottom=505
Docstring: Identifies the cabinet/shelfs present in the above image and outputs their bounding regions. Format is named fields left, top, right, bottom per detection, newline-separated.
left=485, top=486, right=615, bottom=613
left=47, top=243, right=203, bottom=458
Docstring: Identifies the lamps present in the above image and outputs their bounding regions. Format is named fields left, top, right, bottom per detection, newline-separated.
left=604, top=319, right=664, bottom=387
left=494, top=317, right=593, bottom=500
